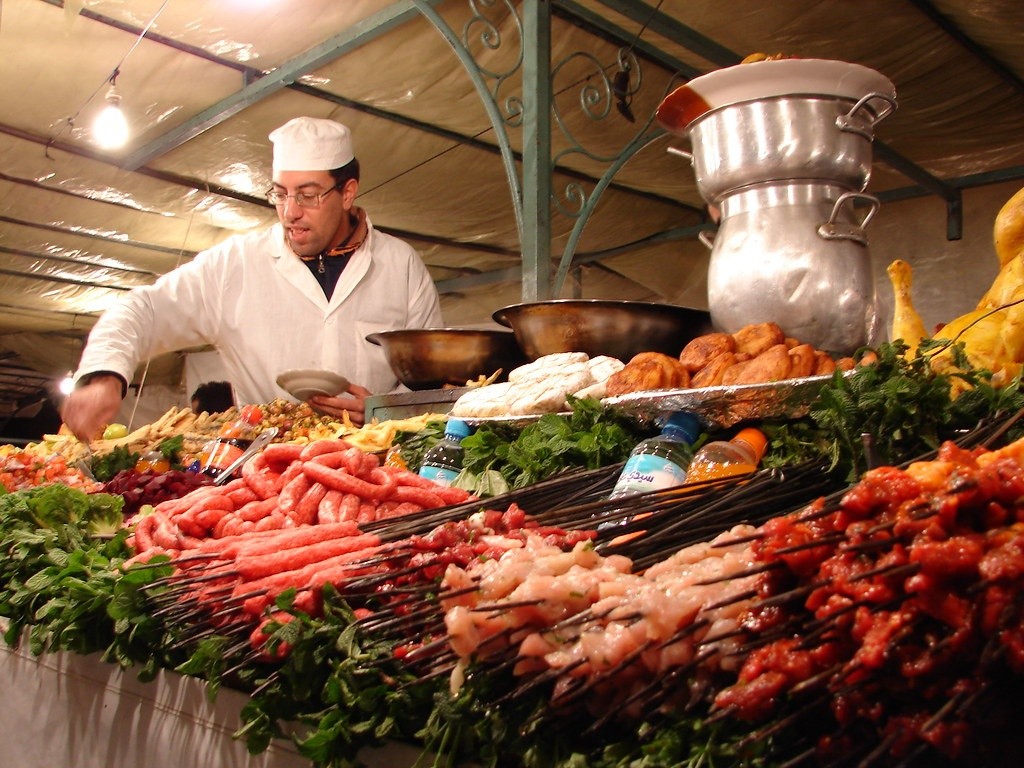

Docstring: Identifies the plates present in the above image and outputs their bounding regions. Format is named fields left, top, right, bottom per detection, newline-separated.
left=276, top=368, right=351, bottom=402
left=655, top=59, right=897, bottom=137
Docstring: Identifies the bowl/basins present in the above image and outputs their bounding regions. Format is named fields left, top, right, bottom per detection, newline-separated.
left=365, top=327, right=515, bottom=391
left=491, top=298, right=710, bottom=365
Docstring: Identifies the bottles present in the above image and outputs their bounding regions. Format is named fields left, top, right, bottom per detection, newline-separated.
left=134, top=450, right=170, bottom=474
left=384, top=443, right=408, bottom=470
left=592, top=412, right=769, bottom=553
left=187, top=422, right=234, bottom=473
left=418, top=419, right=470, bottom=487
left=200, top=405, right=263, bottom=486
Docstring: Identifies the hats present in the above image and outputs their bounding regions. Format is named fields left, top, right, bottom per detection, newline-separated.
left=269, top=117, right=354, bottom=170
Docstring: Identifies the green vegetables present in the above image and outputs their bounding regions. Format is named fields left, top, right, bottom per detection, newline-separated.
left=390, top=336, right=1024, bottom=493
left=0, top=484, right=1024, bottom=768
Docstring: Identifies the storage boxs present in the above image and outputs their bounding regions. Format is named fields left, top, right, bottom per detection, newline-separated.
left=364, top=388, right=466, bottom=424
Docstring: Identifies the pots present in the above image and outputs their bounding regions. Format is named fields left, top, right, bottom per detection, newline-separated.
left=667, top=90, right=896, bottom=355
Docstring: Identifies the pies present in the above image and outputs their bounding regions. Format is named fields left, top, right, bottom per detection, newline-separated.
left=451, top=319, right=882, bottom=417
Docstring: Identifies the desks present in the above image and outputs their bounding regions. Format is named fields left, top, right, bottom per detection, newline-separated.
left=0, top=616, right=452, bottom=768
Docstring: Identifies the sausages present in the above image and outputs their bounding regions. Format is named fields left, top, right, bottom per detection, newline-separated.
left=121, top=438, right=483, bottom=630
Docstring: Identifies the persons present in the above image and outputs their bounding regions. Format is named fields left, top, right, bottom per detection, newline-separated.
left=191, top=381, right=234, bottom=417
left=63, top=116, right=443, bottom=445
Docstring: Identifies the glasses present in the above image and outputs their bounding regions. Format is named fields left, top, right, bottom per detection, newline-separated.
left=264, top=180, right=347, bottom=207
